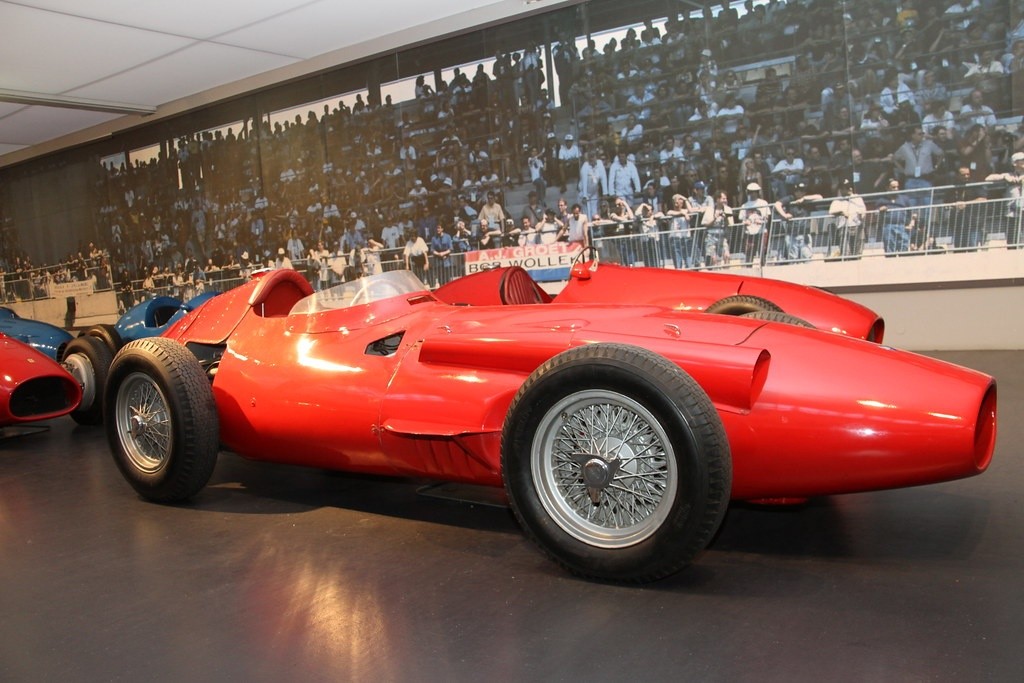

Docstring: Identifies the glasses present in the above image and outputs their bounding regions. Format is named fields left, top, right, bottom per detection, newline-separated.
left=916, top=132, right=925, bottom=135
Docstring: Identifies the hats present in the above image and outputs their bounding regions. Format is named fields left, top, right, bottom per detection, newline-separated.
left=528, top=191, right=536, bottom=196
left=793, top=182, right=808, bottom=190
left=1011, top=152, right=1024, bottom=163
left=839, top=179, right=854, bottom=189
left=241, top=251, right=249, bottom=259
left=545, top=209, right=555, bottom=213
left=444, top=178, right=453, bottom=186
left=547, top=133, right=554, bottom=139
left=153, top=267, right=159, bottom=273
left=430, top=175, right=437, bottom=182
left=565, top=134, right=574, bottom=140
left=694, top=181, right=705, bottom=189
left=278, top=248, right=286, bottom=255
left=414, top=180, right=422, bottom=185
left=701, top=49, right=712, bottom=56
left=746, top=182, right=761, bottom=191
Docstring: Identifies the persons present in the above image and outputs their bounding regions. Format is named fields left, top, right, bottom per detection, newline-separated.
left=0, top=0, right=1024, bottom=316
